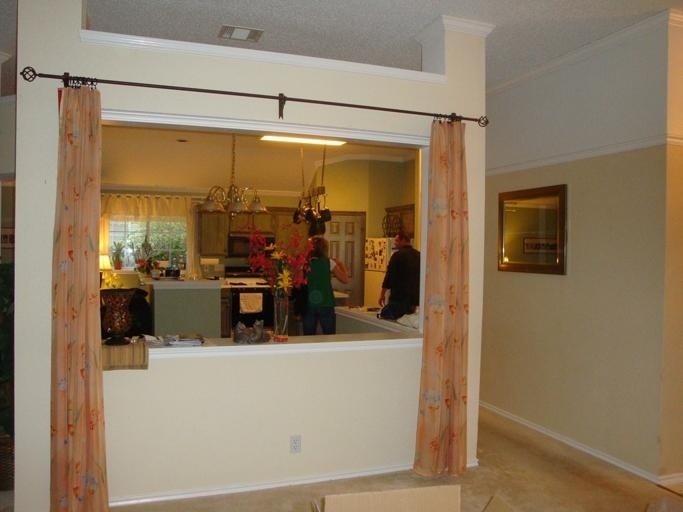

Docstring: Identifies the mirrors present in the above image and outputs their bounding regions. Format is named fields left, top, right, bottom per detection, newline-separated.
left=497, top=183, right=568, bottom=276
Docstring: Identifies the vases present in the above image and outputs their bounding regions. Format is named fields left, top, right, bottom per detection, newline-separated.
left=273, top=296, right=291, bottom=342
left=101, top=289, right=136, bottom=346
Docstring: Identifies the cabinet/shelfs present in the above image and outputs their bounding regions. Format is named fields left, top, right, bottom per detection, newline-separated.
left=149, top=284, right=221, bottom=339
left=195, top=204, right=229, bottom=256
left=229, top=207, right=276, bottom=235
left=276, top=207, right=311, bottom=257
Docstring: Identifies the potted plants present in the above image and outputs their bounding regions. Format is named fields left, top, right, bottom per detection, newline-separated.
left=112, top=242, right=125, bottom=270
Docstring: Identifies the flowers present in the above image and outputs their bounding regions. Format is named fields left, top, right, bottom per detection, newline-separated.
left=246, top=221, right=319, bottom=334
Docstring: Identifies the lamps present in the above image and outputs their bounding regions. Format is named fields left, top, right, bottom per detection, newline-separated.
left=99, top=255, right=111, bottom=280
left=292, top=144, right=331, bottom=224
left=197, top=132, right=268, bottom=218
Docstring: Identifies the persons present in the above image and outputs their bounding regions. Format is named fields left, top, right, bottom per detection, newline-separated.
left=377, top=231, right=420, bottom=320
left=294, top=236, right=349, bottom=337
